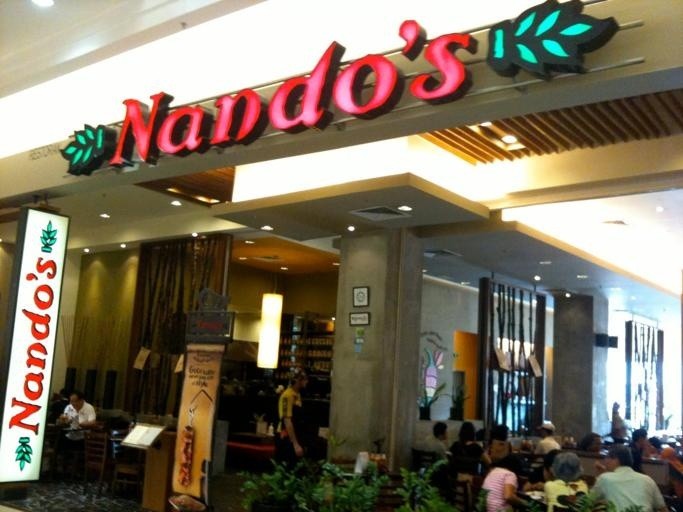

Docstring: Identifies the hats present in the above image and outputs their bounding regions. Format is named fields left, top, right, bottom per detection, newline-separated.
left=550, top=452, right=582, bottom=480
left=537, top=423, right=555, bottom=431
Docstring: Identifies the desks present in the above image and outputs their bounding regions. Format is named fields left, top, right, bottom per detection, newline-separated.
left=44, top=422, right=70, bottom=483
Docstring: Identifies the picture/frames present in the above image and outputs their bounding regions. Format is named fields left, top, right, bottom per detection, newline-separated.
left=349, top=311, right=370, bottom=326
left=350, top=286, right=370, bottom=308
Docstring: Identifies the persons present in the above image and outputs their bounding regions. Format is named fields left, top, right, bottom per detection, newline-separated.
left=42, top=385, right=97, bottom=483
left=214, top=368, right=289, bottom=396
left=275, top=369, right=307, bottom=469
left=415, top=413, right=683, bottom=512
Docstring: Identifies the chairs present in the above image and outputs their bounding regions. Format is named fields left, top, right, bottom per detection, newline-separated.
left=79, top=430, right=113, bottom=501
left=333, top=444, right=682, bottom=511
left=109, top=434, right=146, bottom=503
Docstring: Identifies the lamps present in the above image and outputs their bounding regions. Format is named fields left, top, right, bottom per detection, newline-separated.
left=254, top=258, right=285, bottom=371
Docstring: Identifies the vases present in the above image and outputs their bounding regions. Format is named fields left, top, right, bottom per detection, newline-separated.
left=63, top=365, right=77, bottom=394
left=101, top=369, right=119, bottom=411
left=83, top=367, right=98, bottom=405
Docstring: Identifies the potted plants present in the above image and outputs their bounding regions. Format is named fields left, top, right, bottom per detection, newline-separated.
left=417, top=380, right=448, bottom=422
left=449, top=388, right=471, bottom=421
left=236, top=467, right=298, bottom=512
